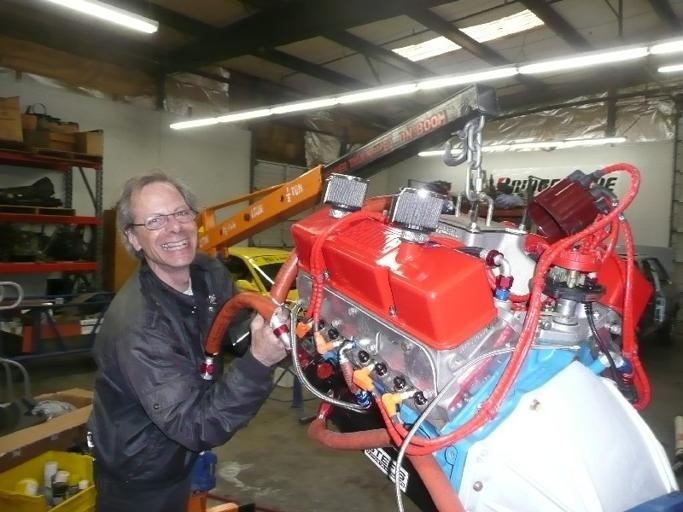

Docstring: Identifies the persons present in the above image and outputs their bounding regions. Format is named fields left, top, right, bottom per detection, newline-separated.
left=85, top=169, right=289, bottom=512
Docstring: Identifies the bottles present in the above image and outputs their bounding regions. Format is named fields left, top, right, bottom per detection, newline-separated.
left=41, top=456, right=90, bottom=507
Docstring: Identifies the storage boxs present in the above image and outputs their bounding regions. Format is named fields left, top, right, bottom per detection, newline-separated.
left=0, top=382, right=97, bottom=510
left=0, top=94, right=105, bottom=160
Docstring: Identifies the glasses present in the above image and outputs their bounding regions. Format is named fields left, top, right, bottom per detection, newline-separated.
left=128, top=207, right=199, bottom=231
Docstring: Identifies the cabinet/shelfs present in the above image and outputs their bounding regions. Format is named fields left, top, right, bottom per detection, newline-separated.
left=0, top=143, right=104, bottom=367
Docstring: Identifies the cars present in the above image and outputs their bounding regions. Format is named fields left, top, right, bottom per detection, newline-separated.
left=228, top=246, right=307, bottom=318
